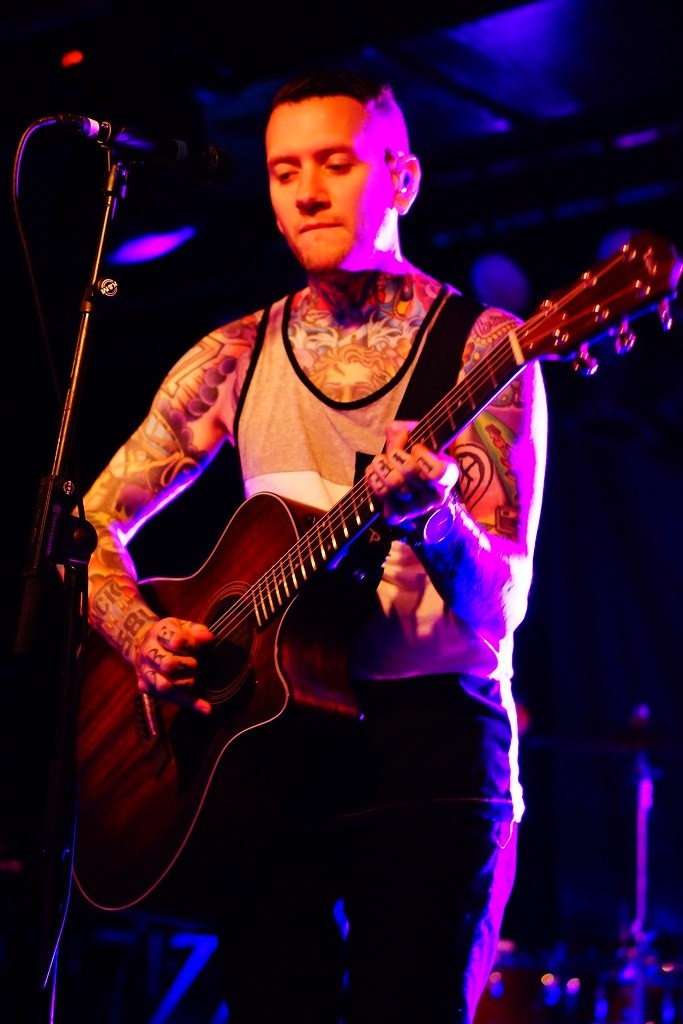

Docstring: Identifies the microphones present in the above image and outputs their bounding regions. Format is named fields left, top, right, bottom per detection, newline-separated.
left=62, top=113, right=219, bottom=174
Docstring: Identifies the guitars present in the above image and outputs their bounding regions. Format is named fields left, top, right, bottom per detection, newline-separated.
left=70, top=224, right=683, bottom=917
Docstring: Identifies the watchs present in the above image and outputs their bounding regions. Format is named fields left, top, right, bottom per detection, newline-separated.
left=405, top=494, right=464, bottom=550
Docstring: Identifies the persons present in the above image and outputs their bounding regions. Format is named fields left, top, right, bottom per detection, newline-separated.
left=52, top=54, right=551, bottom=1024
